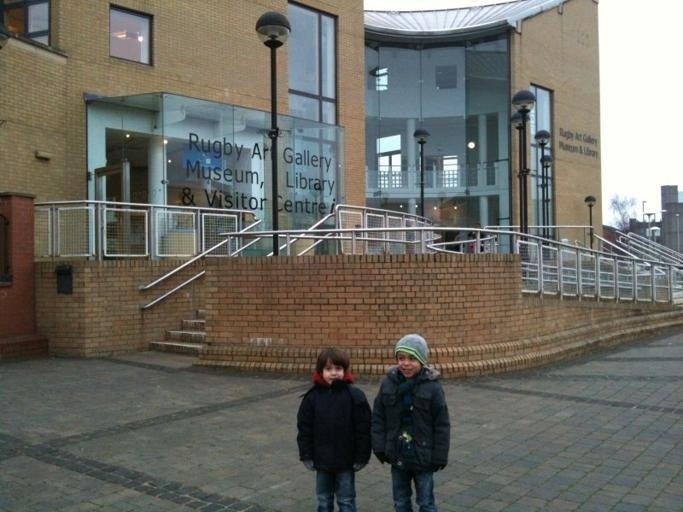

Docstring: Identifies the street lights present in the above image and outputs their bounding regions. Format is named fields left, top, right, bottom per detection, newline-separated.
left=509, top=89, right=554, bottom=281
left=413, top=126, right=432, bottom=223
left=255, top=10, right=293, bottom=256
left=584, top=195, right=597, bottom=261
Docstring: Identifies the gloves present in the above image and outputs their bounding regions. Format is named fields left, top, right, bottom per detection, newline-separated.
left=353, top=463, right=366, bottom=471
left=374, top=453, right=391, bottom=465
left=432, top=463, right=447, bottom=471
left=304, top=459, right=316, bottom=471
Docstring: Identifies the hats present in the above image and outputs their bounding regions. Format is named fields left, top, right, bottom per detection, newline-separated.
left=394, top=334, right=428, bottom=367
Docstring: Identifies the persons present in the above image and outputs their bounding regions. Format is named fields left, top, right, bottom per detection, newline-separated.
left=469, top=223, right=484, bottom=252
left=372, top=334, right=450, bottom=512
left=297, top=348, right=372, bottom=512
